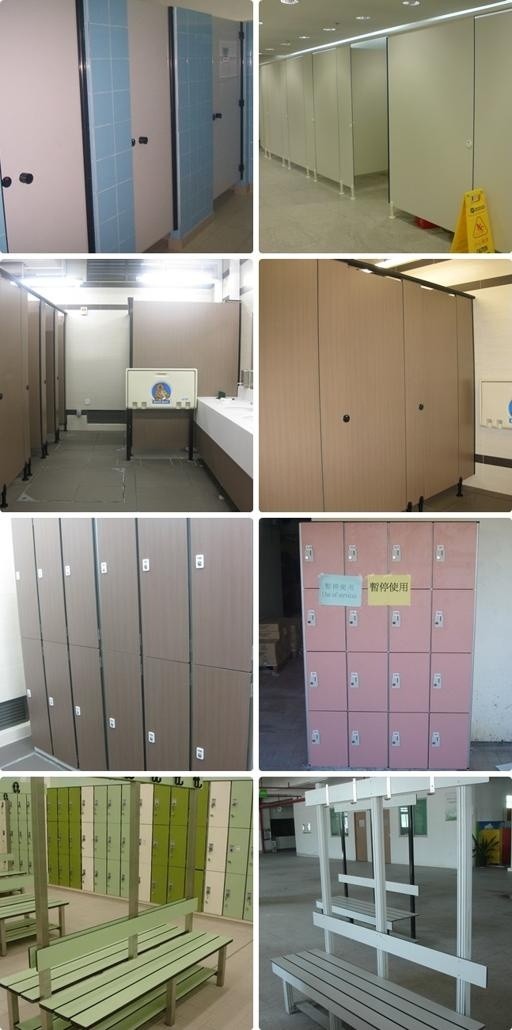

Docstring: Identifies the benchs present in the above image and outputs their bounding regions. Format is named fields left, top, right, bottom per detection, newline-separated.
left=0, top=869, right=234, bottom=1030
left=316, top=895, right=419, bottom=949
left=271, top=914, right=492, bottom=1030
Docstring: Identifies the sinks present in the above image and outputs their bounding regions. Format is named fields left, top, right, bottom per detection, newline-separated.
left=222, top=405, right=254, bottom=419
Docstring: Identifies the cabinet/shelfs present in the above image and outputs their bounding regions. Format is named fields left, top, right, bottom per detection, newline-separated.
left=0, top=781, right=253, bottom=923
left=298, top=521, right=477, bottom=769
left=11, top=516, right=251, bottom=770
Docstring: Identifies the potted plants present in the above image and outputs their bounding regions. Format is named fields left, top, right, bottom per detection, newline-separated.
left=471, top=834, right=501, bottom=868
